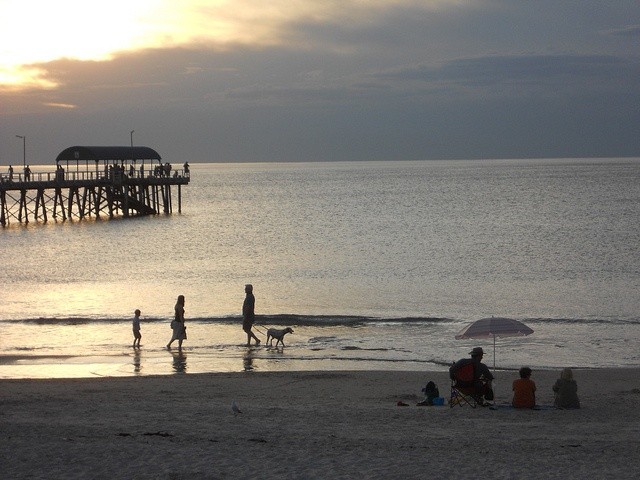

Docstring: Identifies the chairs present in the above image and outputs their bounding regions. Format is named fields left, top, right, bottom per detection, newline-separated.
left=451, top=361, right=479, bottom=408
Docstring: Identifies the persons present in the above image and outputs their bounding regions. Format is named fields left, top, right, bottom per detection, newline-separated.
left=154, top=163, right=172, bottom=178
left=183, top=161, right=190, bottom=177
left=459, top=347, right=502, bottom=410
left=104, top=165, right=108, bottom=179
left=166, top=295, right=187, bottom=349
left=140, top=165, right=144, bottom=178
left=132, top=309, right=142, bottom=347
left=128, top=165, right=135, bottom=177
left=59, top=165, right=64, bottom=180
left=552, top=368, right=580, bottom=410
left=242, top=284, right=261, bottom=346
left=8, top=165, right=13, bottom=181
left=23, top=165, right=31, bottom=182
left=512, top=367, right=536, bottom=408
left=109, top=165, right=124, bottom=179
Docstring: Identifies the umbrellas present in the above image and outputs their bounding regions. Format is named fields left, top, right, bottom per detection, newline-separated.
left=455, top=315, right=533, bottom=372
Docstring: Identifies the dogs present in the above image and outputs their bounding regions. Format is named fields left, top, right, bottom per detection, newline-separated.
left=266, top=328, right=294, bottom=347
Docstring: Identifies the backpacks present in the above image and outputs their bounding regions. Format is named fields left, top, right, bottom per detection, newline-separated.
left=421, top=381, right=439, bottom=405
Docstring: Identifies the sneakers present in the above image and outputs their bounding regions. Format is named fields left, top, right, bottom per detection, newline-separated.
left=484, top=400, right=496, bottom=410
left=481, top=401, right=489, bottom=405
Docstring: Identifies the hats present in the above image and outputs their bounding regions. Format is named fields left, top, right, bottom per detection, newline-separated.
left=561, top=368, right=574, bottom=381
left=469, top=347, right=487, bottom=355
